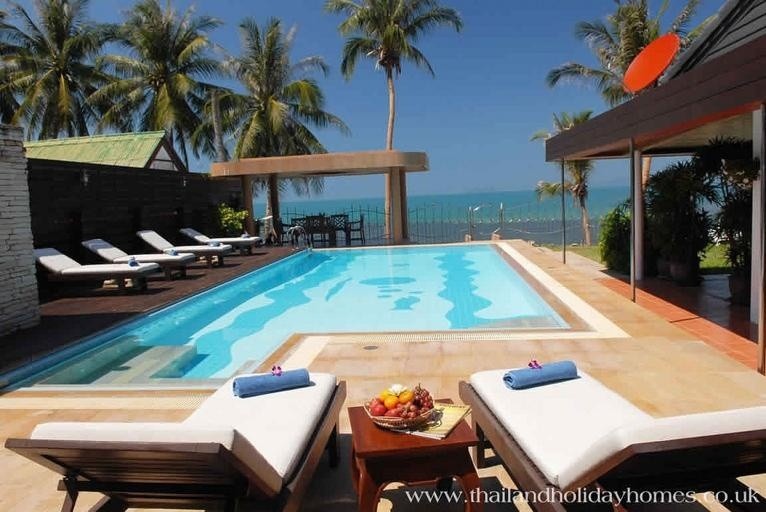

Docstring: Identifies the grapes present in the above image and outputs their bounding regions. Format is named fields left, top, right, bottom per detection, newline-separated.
left=397, top=382, right=433, bottom=418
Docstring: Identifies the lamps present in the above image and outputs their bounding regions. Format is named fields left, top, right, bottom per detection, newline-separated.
left=80, top=168, right=91, bottom=189
left=178, top=174, right=188, bottom=188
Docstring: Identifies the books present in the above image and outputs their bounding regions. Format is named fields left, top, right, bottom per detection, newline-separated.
left=391, top=402, right=472, bottom=440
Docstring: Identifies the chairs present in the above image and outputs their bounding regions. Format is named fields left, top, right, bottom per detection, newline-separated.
left=33, top=247, right=160, bottom=294
left=180, top=226, right=262, bottom=257
left=280, top=213, right=368, bottom=246
left=5, top=372, right=347, bottom=512
left=459, top=365, right=766, bottom=511
left=136, top=229, right=231, bottom=267
left=80, top=237, right=196, bottom=282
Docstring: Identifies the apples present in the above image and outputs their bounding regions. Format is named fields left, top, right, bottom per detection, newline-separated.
left=370, top=397, right=387, bottom=416
left=385, top=408, right=400, bottom=416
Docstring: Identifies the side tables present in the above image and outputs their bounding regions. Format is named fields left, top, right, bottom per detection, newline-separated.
left=348, top=397, right=486, bottom=512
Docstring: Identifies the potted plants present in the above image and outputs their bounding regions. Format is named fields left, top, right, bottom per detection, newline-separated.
left=215, top=201, right=249, bottom=250
left=598, top=135, right=759, bottom=300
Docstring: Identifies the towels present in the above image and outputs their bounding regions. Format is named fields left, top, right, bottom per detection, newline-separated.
left=129, top=257, right=135, bottom=266
left=503, top=360, right=577, bottom=390
left=170, top=249, right=178, bottom=256
left=241, top=234, right=251, bottom=238
left=208, top=242, right=220, bottom=247
left=232, top=365, right=310, bottom=398
